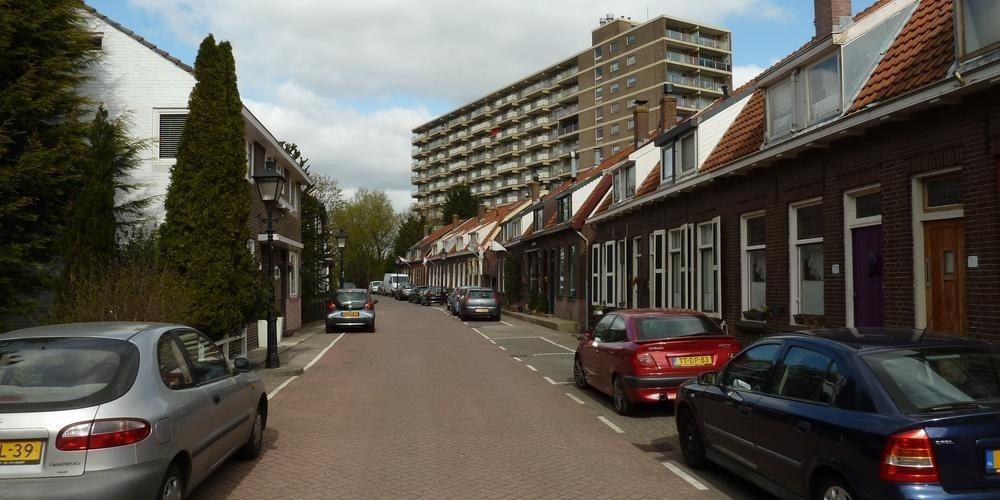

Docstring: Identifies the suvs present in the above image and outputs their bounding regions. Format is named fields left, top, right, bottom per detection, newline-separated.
left=458, top=288, right=503, bottom=321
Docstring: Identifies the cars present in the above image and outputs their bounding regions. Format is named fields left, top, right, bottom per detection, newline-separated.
left=572, top=307, right=741, bottom=414
left=677, top=328, right=999, bottom=498
left=0, top=320, right=269, bottom=499
left=325, top=288, right=380, bottom=335
left=342, top=282, right=355, bottom=289
left=368, top=279, right=381, bottom=294
left=394, top=283, right=479, bottom=315
left=378, top=281, right=384, bottom=294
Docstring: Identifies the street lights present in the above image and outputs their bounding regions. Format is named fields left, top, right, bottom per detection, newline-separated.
left=250, top=156, right=286, bottom=366
left=335, top=229, right=349, bottom=287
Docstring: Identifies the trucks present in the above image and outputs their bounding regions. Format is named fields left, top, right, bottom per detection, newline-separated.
left=384, top=273, right=410, bottom=295
